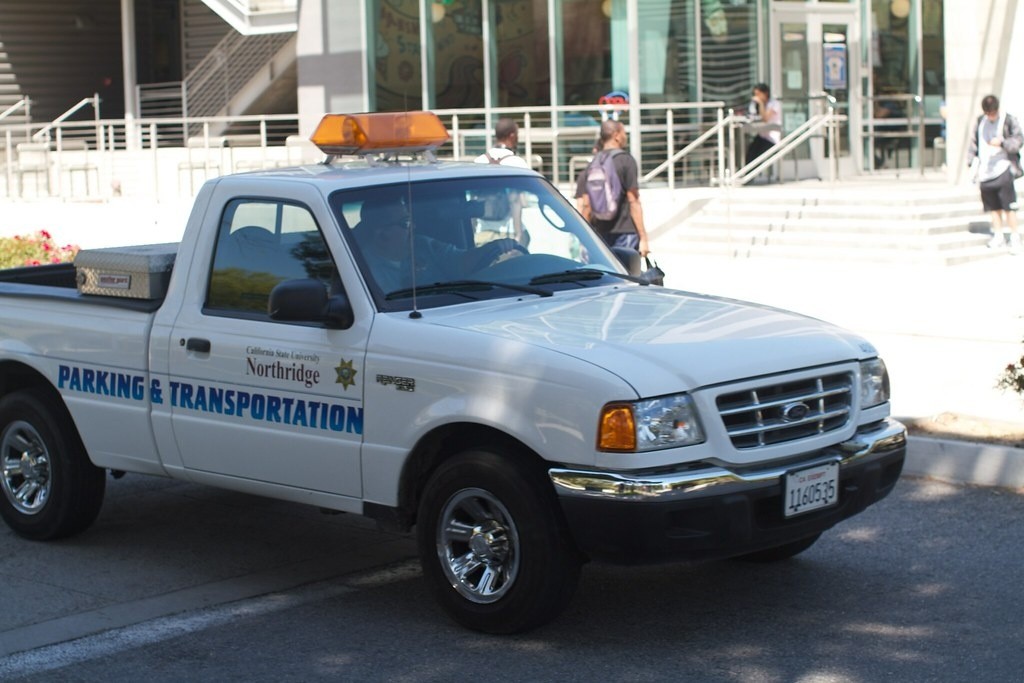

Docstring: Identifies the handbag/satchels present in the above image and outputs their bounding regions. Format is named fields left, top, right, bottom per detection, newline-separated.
left=638, top=248, right=664, bottom=287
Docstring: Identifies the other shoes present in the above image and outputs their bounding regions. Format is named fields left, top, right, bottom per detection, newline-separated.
left=1010, top=240, right=1021, bottom=254
left=988, top=236, right=1006, bottom=248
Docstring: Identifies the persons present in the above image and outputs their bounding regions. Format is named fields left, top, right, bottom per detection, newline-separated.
left=470, top=118, right=529, bottom=269
left=966, top=95, right=1024, bottom=255
left=572, top=120, right=650, bottom=276
left=718, top=83, right=783, bottom=188
left=358, top=192, right=518, bottom=306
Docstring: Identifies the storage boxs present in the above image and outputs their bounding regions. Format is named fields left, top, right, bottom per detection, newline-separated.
left=74, top=241, right=181, bottom=300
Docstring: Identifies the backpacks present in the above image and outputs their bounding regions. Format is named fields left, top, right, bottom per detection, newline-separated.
left=470, top=152, right=513, bottom=222
left=582, top=146, right=625, bottom=222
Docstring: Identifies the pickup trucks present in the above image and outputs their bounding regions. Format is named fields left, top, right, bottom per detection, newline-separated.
left=0, top=109, right=910, bottom=638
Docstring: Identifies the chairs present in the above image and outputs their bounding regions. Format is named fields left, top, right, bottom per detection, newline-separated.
left=219, top=226, right=274, bottom=312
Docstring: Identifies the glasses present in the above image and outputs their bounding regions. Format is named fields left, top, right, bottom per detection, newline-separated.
left=378, top=214, right=411, bottom=229
